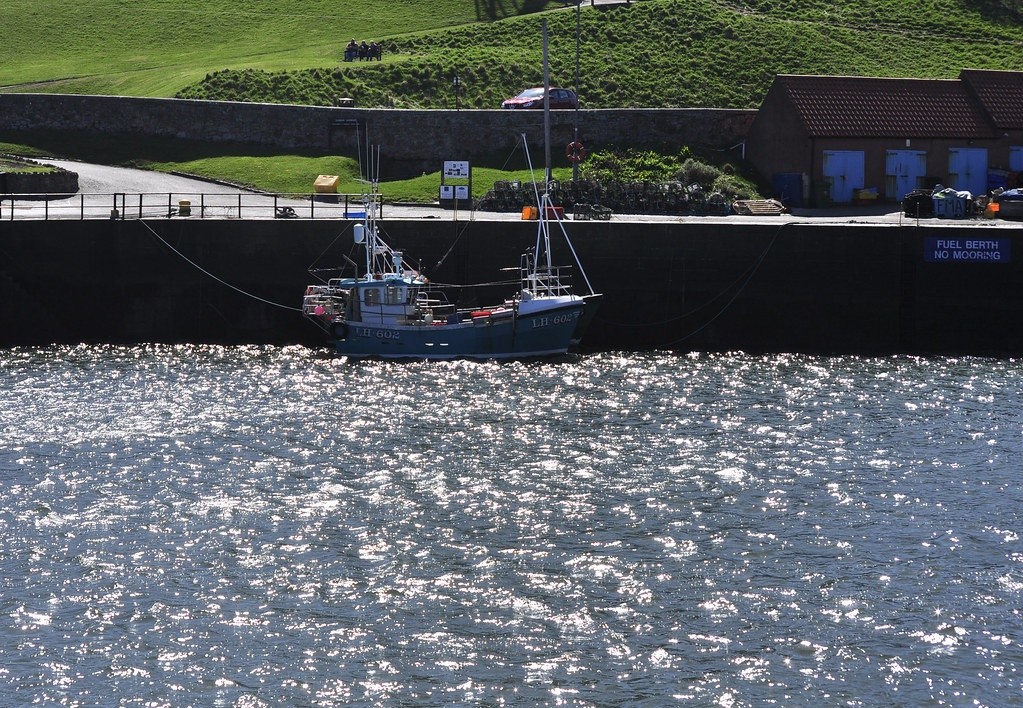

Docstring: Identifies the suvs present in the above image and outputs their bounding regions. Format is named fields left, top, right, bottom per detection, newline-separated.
left=501, top=87, right=579, bottom=109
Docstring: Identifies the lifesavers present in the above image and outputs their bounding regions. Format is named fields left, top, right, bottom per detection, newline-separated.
left=566, top=141, right=585, bottom=161
left=306, top=288, right=319, bottom=304
left=330, top=321, right=348, bottom=339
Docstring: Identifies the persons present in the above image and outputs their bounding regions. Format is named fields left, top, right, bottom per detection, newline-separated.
left=366, top=40, right=378, bottom=61
left=359, top=40, right=368, bottom=61
left=346, top=38, right=358, bottom=61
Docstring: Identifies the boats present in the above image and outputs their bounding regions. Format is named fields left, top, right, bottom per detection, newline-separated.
left=303, top=121, right=604, bottom=363
